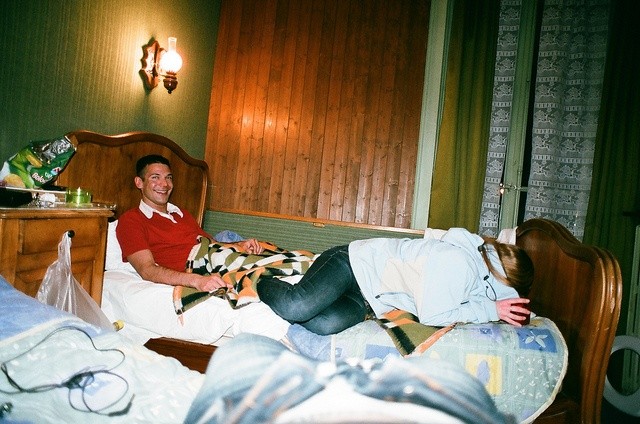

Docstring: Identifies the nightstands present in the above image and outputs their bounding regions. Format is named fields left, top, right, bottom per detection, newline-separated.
left=1, top=195, right=115, bottom=318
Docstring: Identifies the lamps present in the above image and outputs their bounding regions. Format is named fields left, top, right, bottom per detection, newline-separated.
left=139, top=35, right=182, bottom=96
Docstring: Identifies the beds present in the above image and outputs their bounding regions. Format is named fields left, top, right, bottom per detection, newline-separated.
left=60, top=127, right=623, bottom=424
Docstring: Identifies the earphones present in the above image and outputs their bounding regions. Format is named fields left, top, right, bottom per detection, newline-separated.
left=1, top=402, right=14, bottom=421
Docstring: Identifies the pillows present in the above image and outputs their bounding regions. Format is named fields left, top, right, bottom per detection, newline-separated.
left=103, top=214, right=140, bottom=276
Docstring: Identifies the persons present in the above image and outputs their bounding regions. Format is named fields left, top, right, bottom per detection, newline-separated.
left=257, top=228, right=534, bottom=337
left=115, top=155, right=265, bottom=295
